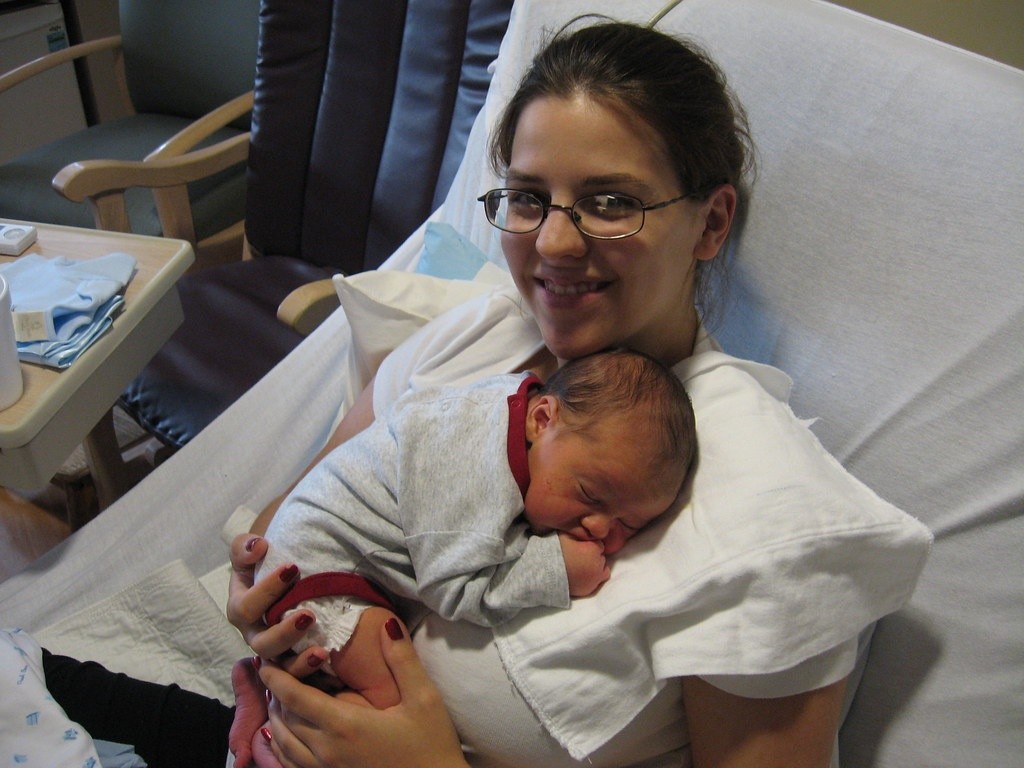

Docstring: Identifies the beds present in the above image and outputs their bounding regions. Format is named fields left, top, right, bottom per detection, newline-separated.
left=1, top=1, right=1022, bottom=768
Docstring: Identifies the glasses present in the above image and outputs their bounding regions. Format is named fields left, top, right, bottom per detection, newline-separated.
left=478, top=187, right=689, bottom=240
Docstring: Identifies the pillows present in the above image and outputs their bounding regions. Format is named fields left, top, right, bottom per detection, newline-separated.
left=322, top=270, right=496, bottom=453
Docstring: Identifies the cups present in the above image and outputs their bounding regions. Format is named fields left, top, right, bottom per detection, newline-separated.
left=0, top=274, right=23, bottom=412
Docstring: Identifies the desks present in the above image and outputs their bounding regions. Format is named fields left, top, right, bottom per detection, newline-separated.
left=0, top=218, right=195, bottom=499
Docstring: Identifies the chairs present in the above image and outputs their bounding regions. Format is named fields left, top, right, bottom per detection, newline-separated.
left=51, top=0, right=515, bottom=513
left=1, top=0, right=261, bottom=530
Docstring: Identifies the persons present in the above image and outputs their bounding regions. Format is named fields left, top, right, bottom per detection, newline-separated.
left=226, top=24, right=882, bottom=768
